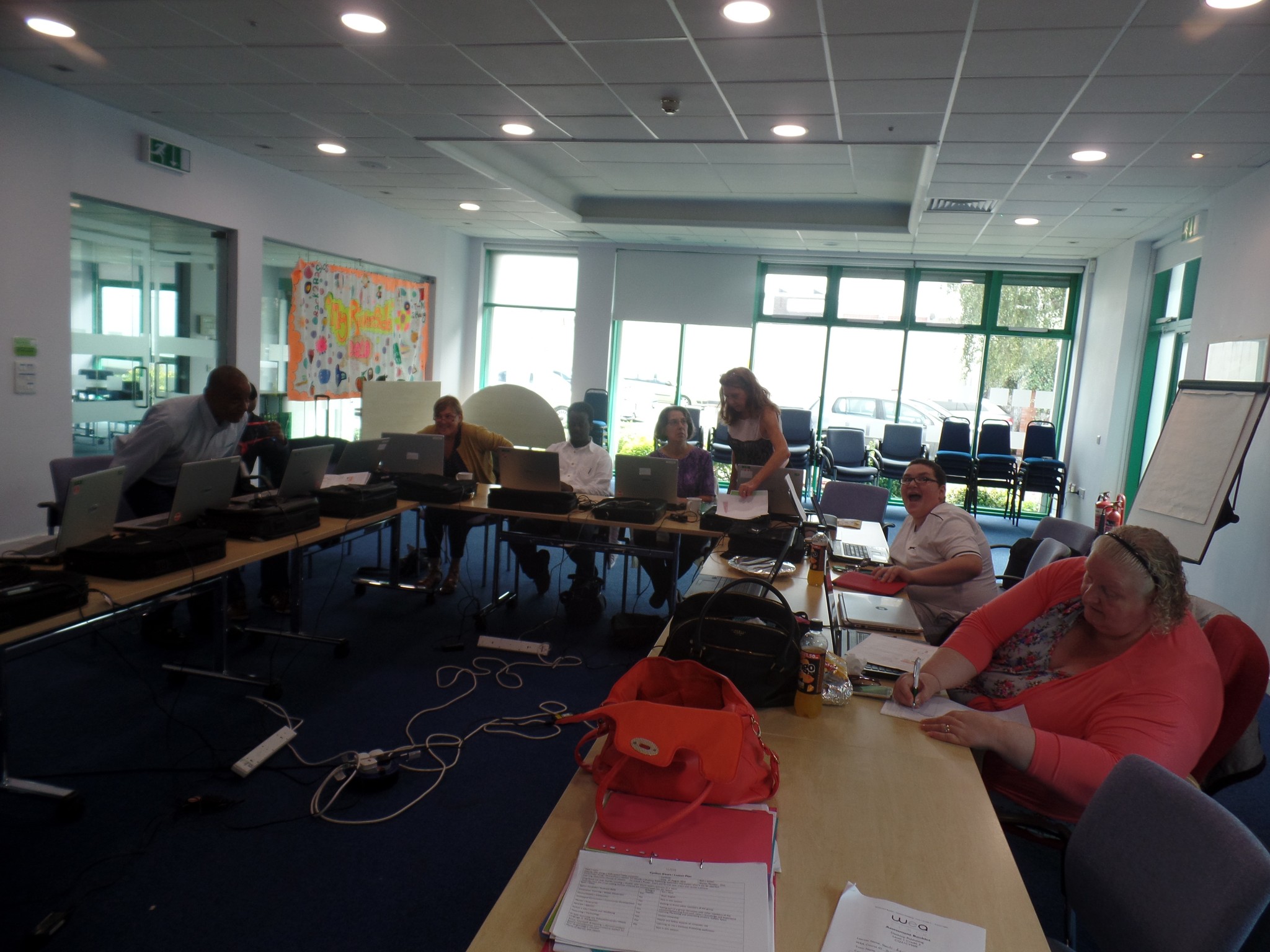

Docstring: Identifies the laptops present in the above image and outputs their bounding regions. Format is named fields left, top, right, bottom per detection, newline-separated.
left=614, top=455, right=679, bottom=502
left=737, top=464, right=804, bottom=516
left=334, top=439, right=386, bottom=479
left=114, top=454, right=240, bottom=532
left=808, top=486, right=895, bottom=565
left=784, top=473, right=840, bottom=532
left=382, top=431, right=444, bottom=477
left=230, top=442, right=333, bottom=504
left=498, top=447, right=563, bottom=490
left=1, top=463, right=125, bottom=560
left=838, top=590, right=923, bottom=634
left=822, top=577, right=932, bottom=684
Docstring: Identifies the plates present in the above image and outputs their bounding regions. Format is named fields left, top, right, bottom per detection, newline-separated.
left=728, top=556, right=796, bottom=579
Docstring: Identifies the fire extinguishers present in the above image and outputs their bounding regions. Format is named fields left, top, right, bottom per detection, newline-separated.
left=1094, top=492, right=1114, bottom=535
left=1104, top=500, right=1124, bottom=535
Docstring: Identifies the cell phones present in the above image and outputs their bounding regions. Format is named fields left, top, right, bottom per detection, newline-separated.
left=851, top=684, right=894, bottom=700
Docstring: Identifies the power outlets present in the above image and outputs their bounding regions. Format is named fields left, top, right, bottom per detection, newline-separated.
left=1068, top=483, right=1085, bottom=499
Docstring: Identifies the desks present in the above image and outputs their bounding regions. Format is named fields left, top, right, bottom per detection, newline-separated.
left=1, top=480, right=1052, bottom=951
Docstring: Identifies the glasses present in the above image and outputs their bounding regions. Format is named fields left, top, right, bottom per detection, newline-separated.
left=900, top=477, right=938, bottom=485
left=719, top=373, right=739, bottom=379
left=433, top=414, right=458, bottom=422
left=665, top=419, right=688, bottom=427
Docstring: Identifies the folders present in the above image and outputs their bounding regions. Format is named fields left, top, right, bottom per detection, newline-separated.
left=540, top=789, right=778, bottom=952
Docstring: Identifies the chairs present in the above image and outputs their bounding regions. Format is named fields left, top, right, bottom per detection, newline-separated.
left=584, top=388, right=611, bottom=453
left=707, top=411, right=733, bottom=465
left=653, top=407, right=704, bottom=451
left=874, top=423, right=930, bottom=486
left=933, top=416, right=973, bottom=513
left=996, top=537, right=1070, bottom=595
left=37, top=455, right=114, bottom=536
left=816, top=426, right=885, bottom=502
left=966, top=418, right=1017, bottom=526
left=989, top=517, right=1098, bottom=595
left=72, top=368, right=143, bottom=447
left=1008, top=421, right=1067, bottom=526
left=1192, top=593, right=1270, bottom=799
left=1046, top=754, right=1269, bottom=952
left=777, top=405, right=815, bottom=503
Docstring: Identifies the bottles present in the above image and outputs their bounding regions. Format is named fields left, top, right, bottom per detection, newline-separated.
left=794, top=619, right=829, bottom=718
left=807, top=526, right=829, bottom=585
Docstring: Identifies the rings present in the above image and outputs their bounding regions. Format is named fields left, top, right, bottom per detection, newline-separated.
left=944, top=724, right=951, bottom=734
left=743, top=491, right=747, bottom=494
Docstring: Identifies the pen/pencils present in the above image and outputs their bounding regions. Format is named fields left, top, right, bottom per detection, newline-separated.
left=911, top=657, right=921, bottom=711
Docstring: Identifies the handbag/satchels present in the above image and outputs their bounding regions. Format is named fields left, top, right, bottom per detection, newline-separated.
left=719, top=521, right=806, bottom=564
left=554, top=654, right=781, bottom=842
left=658, top=575, right=800, bottom=709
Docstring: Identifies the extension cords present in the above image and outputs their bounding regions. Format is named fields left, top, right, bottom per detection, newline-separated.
left=476, top=637, right=553, bottom=658
left=231, top=726, right=299, bottom=779
left=347, top=748, right=396, bottom=781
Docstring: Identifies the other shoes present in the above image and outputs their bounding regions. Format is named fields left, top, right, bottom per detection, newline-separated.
left=415, top=567, right=442, bottom=588
left=649, top=591, right=666, bottom=609
left=440, top=570, right=460, bottom=594
left=534, top=548, right=552, bottom=595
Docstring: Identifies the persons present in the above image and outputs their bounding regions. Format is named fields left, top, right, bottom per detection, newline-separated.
left=223, top=374, right=298, bottom=624
left=404, top=391, right=517, bottom=594
left=720, top=369, right=792, bottom=499
left=632, top=407, right=722, bottom=621
left=897, top=523, right=1224, bottom=873
left=869, top=457, right=1002, bottom=648
left=501, top=402, right=615, bottom=599
left=98, top=364, right=251, bottom=660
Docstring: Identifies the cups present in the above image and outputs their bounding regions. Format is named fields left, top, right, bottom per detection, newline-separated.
left=456, top=472, right=473, bottom=481
left=687, top=497, right=702, bottom=516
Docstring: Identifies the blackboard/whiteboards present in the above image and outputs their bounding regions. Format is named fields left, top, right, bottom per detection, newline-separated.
left=1125, top=379, right=1270, bottom=566
